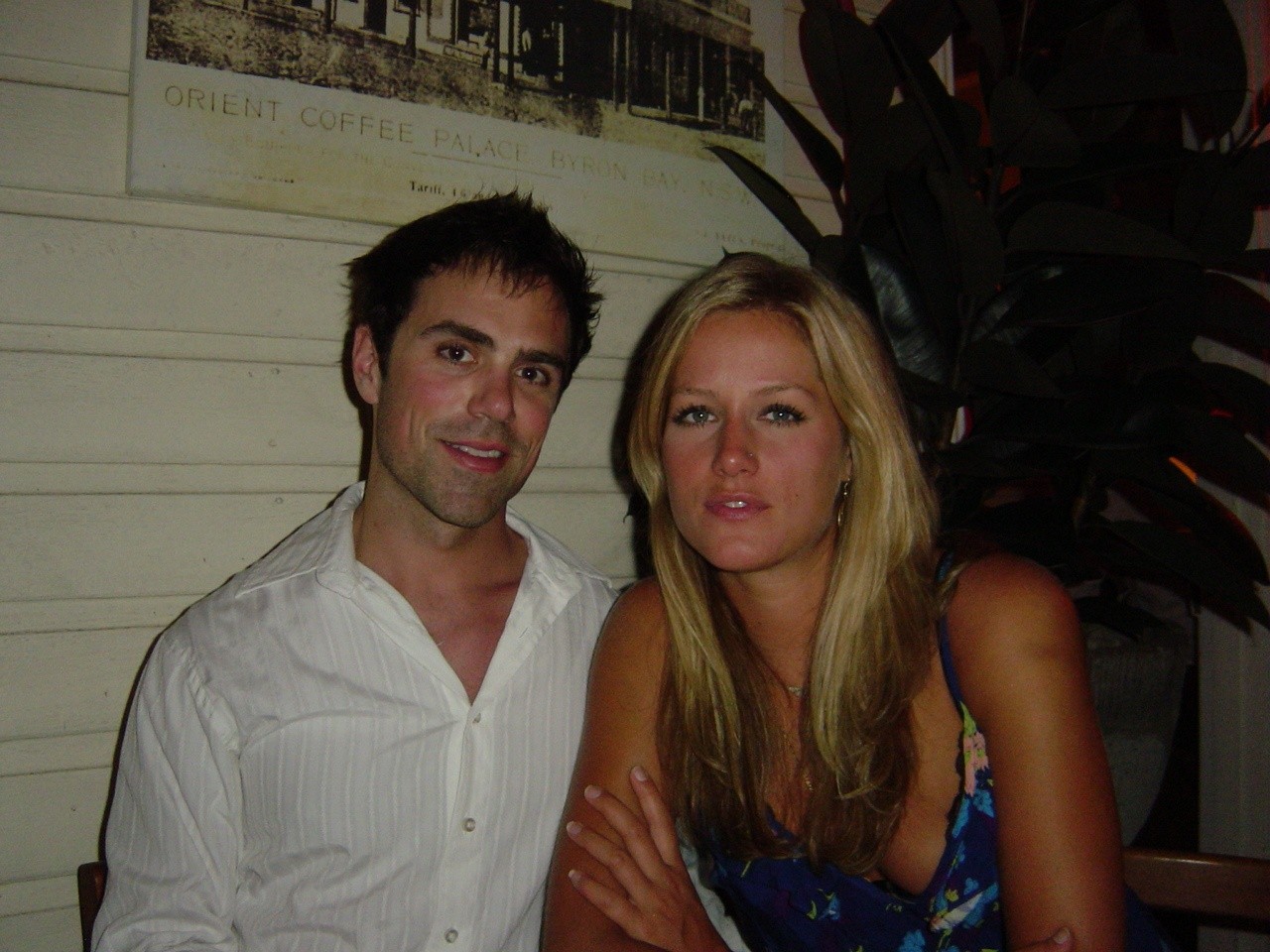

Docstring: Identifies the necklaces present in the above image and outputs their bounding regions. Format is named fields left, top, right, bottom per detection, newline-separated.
left=783, top=730, right=814, bottom=795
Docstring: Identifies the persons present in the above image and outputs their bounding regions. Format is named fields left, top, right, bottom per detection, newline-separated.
left=539, top=252, right=1172, bottom=952
left=90, top=191, right=753, bottom=952
